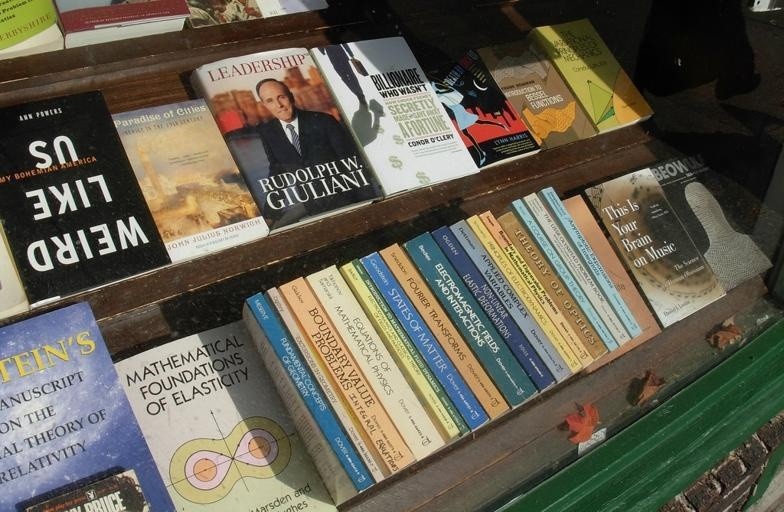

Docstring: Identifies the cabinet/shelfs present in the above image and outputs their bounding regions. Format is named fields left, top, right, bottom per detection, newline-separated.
left=1, top=0, right=781, bottom=512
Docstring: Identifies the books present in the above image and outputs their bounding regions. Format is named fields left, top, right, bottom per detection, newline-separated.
left=1, top=1, right=773, bottom=512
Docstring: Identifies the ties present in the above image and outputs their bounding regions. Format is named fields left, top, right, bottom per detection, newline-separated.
left=286, top=123, right=301, bottom=155
